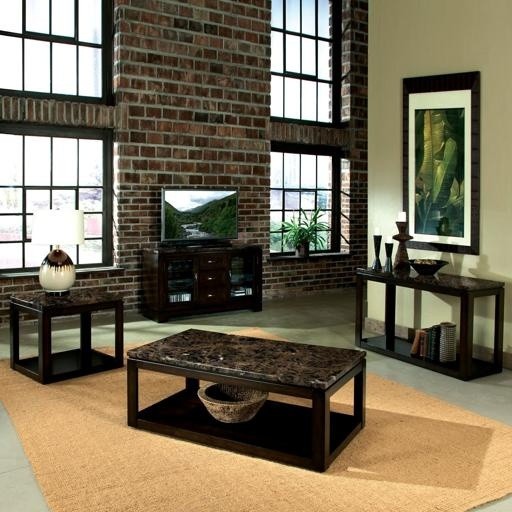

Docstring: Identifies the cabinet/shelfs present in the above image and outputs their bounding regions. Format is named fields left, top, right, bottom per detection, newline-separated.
left=140, top=243, right=264, bottom=324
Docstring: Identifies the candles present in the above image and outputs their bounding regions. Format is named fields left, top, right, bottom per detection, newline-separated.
left=398, top=211, right=407, bottom=222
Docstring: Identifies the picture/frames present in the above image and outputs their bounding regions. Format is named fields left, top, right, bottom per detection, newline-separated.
left=399, top=70, right=481, bottom=256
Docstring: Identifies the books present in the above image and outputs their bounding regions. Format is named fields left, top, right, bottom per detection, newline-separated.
left=408, top=325, right=440, bottom=361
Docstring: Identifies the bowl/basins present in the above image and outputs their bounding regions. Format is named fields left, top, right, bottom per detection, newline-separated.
left=408, top=259, right=449, bottom=277
left=198, top=383, right=269, bottom=422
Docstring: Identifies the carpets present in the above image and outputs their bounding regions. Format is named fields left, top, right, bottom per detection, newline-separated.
left=0, top=329, right=512, bottom=512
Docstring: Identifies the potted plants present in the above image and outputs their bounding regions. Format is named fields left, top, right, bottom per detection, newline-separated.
left=277, top=207, right=332, bottom=257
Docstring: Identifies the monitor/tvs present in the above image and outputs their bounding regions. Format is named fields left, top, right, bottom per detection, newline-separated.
left=161, top=188, right=238, bottom=247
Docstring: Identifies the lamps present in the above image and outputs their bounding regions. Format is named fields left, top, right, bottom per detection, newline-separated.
left=31, top=207, right=85, bottom=297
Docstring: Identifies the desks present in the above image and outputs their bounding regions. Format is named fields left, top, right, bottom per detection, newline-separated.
left=355, top=266, right=505, bottom=381
left=10, top=288, right=124, bottom=385
